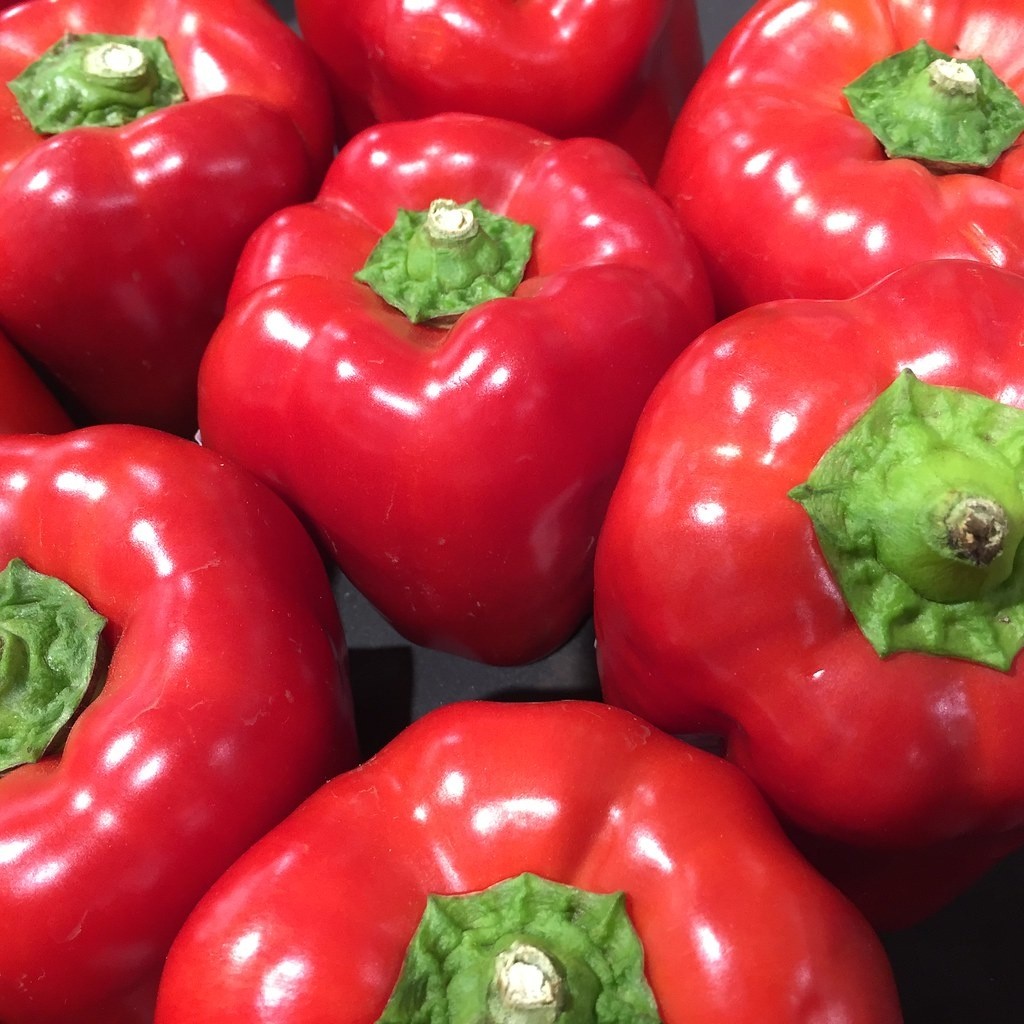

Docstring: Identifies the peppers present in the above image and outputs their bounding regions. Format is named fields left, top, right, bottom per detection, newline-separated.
left=0, top=0, right=1024, bottom=1024
